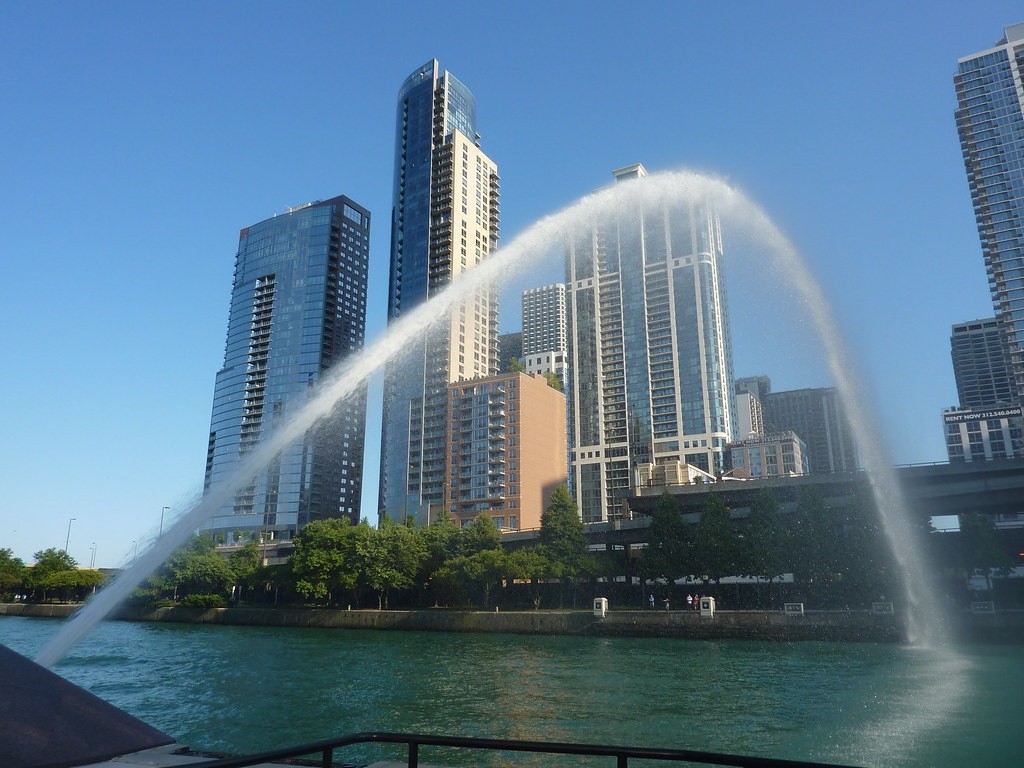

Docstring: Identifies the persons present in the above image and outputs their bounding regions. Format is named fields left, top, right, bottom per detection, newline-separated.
left=663, top=598, right=670, bottom=609
left=649, top=594, right=655, bottom=608
left=686, top=593, right=705, bottom=610
left=879, top=593, right=886, bottom=600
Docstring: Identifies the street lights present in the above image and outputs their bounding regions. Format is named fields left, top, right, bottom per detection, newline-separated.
left=707, top=447, right=713, bottom=475
left=160, top=505, right=170, bottom=537
left=65, top=518, right=77, bottom=551
left=133, top=540, right=138, bottom=557
left=93, top=542, right=96, bottom=568
left=89, top=547, right=93, bottom=565
